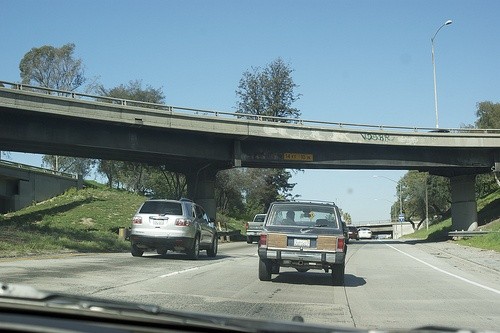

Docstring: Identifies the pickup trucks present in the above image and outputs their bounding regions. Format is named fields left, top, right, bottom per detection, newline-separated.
left=246, top=214, right=267, bottom=244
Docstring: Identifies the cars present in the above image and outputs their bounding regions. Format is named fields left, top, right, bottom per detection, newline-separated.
left=358, top=228, right=372, bottom=240
left=346, top=226, right=360, bottom=240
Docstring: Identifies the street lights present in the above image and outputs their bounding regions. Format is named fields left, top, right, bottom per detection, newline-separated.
left=373, top=176, right=403, bottom=239
left=431, top=20, right=454, bottom=128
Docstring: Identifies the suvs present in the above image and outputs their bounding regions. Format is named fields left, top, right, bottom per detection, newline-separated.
left=258, top=200, right=347, bottom=283
left=131, top=198, right=218, bottom=259
